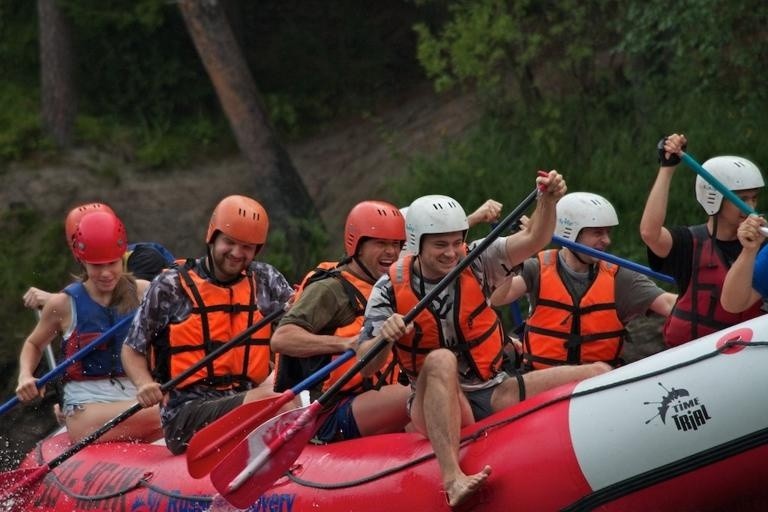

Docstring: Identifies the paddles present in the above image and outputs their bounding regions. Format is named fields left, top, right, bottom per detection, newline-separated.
left=0, top=284, right=300, bottom=512
left=185, top=349, right=356, bottom=480
left=208, top=171, right=548, bottom=510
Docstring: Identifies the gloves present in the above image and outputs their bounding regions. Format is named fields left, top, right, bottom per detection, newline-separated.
left=657, top=135, right=688, bottom=166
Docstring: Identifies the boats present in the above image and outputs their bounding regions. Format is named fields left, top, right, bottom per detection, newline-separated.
left=0, top=315, right=768, bottom=512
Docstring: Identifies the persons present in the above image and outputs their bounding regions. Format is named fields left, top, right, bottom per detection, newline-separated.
left=467, top=199, right=504, bottom=230
left=721, top=213, right=768, bottom=314
left=357, top=168, right=614, bottom=506
left=640, top=132, right=764, bottom=350
left=22, top=204, right=175, bottom=312
left=270, top=199, right=419, bottom=446
left=490, top=191, right=680, bottom=375
left=16, top=212, right=164, bottom=445
left=121, top=195, right=302, bottom=455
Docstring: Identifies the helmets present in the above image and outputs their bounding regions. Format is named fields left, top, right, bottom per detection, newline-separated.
left=72, top=210, right=128, bottom=265
left=65, top=202, right=119, bottom=264
left=554, top=191, right=620, bottom=248
left=345, top=200, right=406, bottom=257
left=695, top=156, right=765, bottom=216
left=404, top=195, right=470, bottom=256
left=397, top=206, right=412, bottom=218
left=205, top=196, right=270, bottom=260
